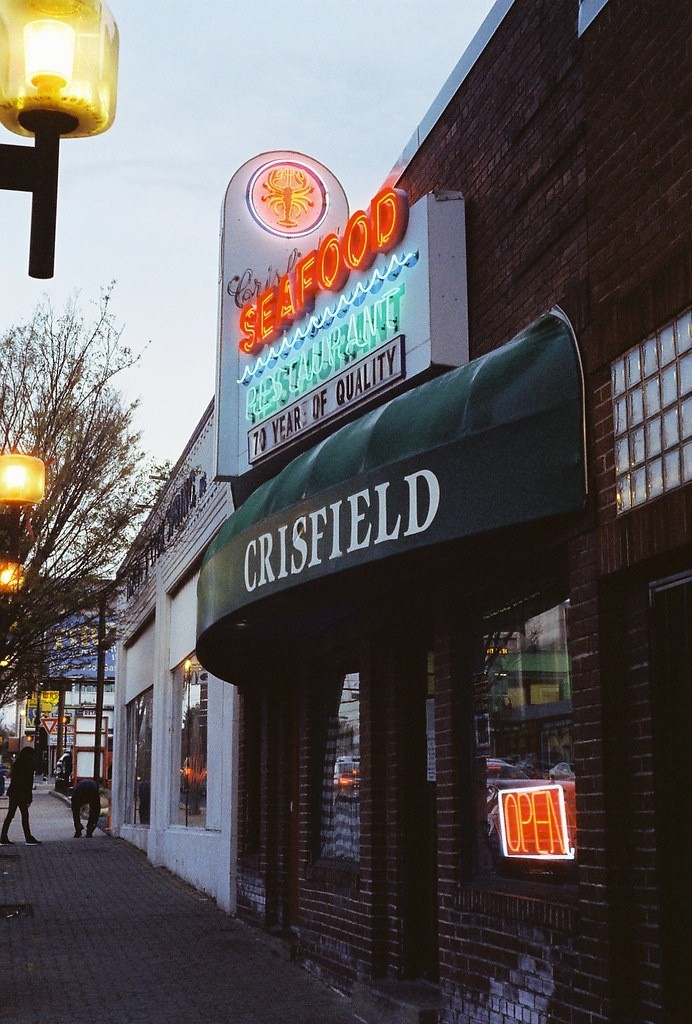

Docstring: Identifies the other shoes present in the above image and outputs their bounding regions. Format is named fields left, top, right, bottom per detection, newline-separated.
left=74, top=823, right=84, bottom=838
left=0, top=840, right=14, bottom=845
left=26, top=839, right=41, bottom=845
left=86, top=833, right=92, bottom=838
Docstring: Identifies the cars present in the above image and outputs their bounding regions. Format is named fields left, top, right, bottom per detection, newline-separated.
left=334, top=756, right=360, bottom=804
left=181, top=757, right=207, bottom=795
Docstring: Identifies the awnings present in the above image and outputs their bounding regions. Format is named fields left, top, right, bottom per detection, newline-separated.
left=195, top=307, right=588, bottom=688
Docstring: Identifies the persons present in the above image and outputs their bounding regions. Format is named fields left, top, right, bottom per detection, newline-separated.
left=70, top=780, right=102, bottom=840
left=0, top=747, right=43, bottom=846
left=137, top=779, right=151, bottom=826
